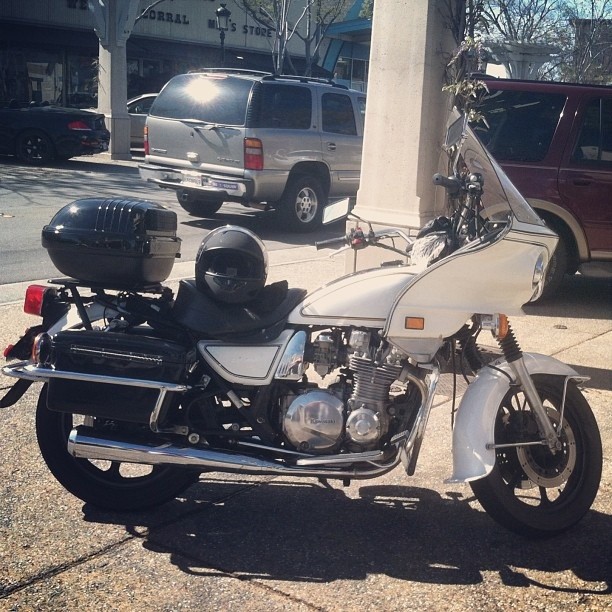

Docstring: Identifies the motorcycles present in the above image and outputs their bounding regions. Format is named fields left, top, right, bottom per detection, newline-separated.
left=4, top=105, right=602, bottom=537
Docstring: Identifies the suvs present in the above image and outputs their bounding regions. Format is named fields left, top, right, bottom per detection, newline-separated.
left=453, top=73, right=612, bottom=301
left=134, top=66, right=366, bottom=230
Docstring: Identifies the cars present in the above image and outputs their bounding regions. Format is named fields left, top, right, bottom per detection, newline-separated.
left=81, top=93, right=160, bottom=148
left=3, top=100, right=110, bottom=167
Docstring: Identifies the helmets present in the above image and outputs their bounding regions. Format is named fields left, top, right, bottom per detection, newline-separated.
left=195, top=224, right=270, bottom=305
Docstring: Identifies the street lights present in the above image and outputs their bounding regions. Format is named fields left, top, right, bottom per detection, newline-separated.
left=216, top=2, right=231, bottom=66
